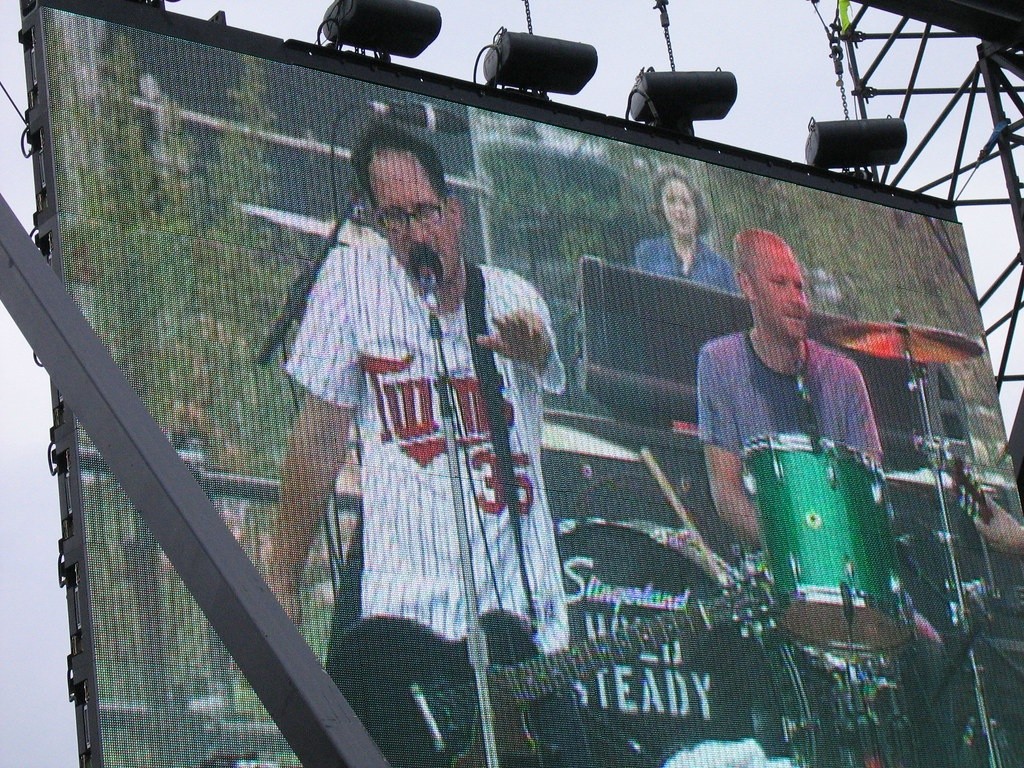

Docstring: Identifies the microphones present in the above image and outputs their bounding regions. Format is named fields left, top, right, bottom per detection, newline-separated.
left=409, top=241, right=445, bottom=338
left=892, top=309, right=917, bottom=388
left=369, top=98, right=469, bottom=133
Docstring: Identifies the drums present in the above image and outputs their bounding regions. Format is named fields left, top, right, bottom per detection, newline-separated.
left=766, top=632, right=937, bottom=768
left=514, top=510, right=846, bottom=768
left=737, top=430, right=920, bottom=660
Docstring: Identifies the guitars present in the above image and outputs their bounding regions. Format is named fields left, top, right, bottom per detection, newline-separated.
left=317, top=568, right=779, bottom=767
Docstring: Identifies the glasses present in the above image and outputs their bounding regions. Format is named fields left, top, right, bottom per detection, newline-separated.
left=371, top=202, right=452, bottom=232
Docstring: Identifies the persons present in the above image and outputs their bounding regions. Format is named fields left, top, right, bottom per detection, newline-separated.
left=972, top=493, right=1024, bottom=555
left=260, top=122, right=588, bottom=704
left=633, top=164, right=739, bottom=292
left=665, top=227, right=969, bottom=768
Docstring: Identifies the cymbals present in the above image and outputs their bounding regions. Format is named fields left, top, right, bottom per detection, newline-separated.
left=884, top=465, right=999, bottom=506
left=826, top=310, right=987, bottom=366
left=540, top=419, right=643, bottom=466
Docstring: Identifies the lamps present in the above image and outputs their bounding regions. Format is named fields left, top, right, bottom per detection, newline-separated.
left=474, top=26, right=598, bottom=101
left=805, top=116, right=907, bottom=180
left=319, top=0, right=442, bottom=59
left=625, top=66, right=740, bottom=136
